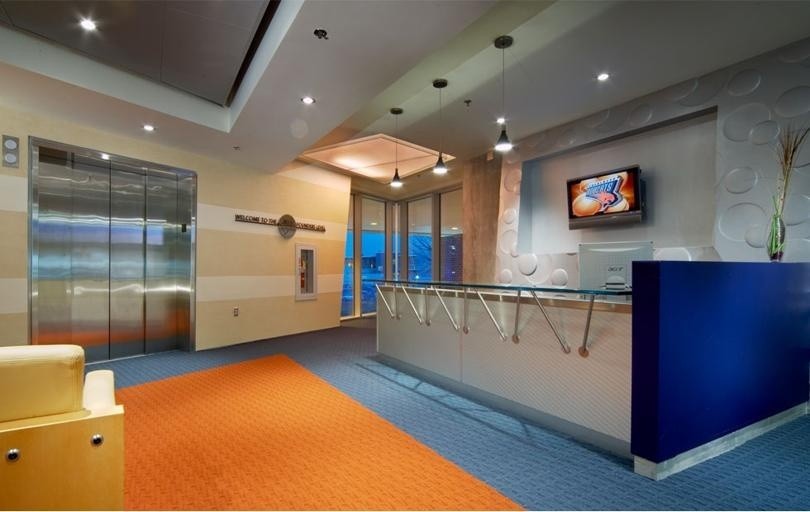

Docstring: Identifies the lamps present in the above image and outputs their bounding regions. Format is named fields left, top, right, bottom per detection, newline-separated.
left=390, top=35, right=515, bottom=189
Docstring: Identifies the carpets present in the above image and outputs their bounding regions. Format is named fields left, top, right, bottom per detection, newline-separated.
left=115, top=352, right=528, bottom=510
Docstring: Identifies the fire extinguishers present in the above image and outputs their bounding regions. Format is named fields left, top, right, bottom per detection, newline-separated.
left=301, top=258, right=306, bottom=288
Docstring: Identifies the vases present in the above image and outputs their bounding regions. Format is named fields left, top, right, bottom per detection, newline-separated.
left=766, top=213, right=788, bottom=262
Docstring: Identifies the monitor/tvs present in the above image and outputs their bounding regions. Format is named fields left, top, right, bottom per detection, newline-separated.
left=565, top=162, right=648, bottom=230
left=576, top=240, right=654, bottom=298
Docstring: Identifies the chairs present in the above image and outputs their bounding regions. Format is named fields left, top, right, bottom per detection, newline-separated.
left=1, top=344, right=124, bottom=511
